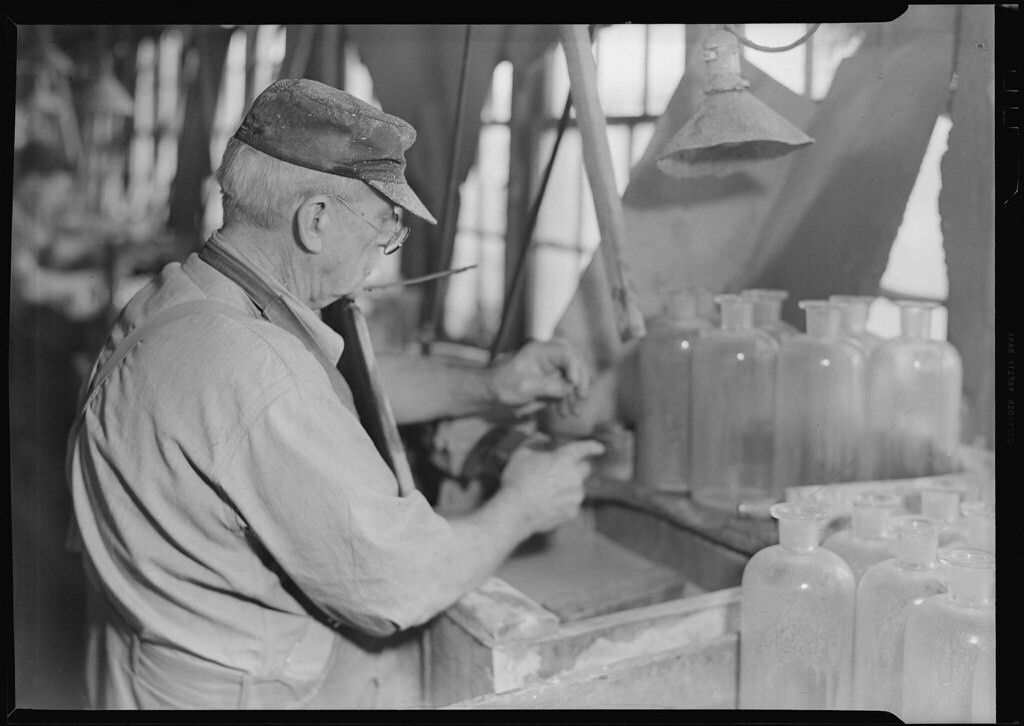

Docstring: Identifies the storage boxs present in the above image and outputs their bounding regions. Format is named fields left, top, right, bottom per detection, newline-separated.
left=422, top=501, right=748, bottom=709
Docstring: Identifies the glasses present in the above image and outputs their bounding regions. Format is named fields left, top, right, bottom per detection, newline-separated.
left=317, top=193, right=410, bottom=255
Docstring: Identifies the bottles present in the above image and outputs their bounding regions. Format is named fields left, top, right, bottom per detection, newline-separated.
left=637, top=286, right=965, bottom=511
left=737, top=491, right=996, bottom=724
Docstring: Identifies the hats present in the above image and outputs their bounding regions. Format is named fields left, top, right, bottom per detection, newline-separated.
left=233, top=78, right=438, bottom=226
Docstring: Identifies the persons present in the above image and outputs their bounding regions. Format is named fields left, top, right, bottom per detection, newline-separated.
left=13, top=144, right=104, bottom=318
left=65, top=79, right=606, bottom=710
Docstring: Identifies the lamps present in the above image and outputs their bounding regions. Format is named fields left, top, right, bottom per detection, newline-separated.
left=656, top=21, right=815, bottom=176
